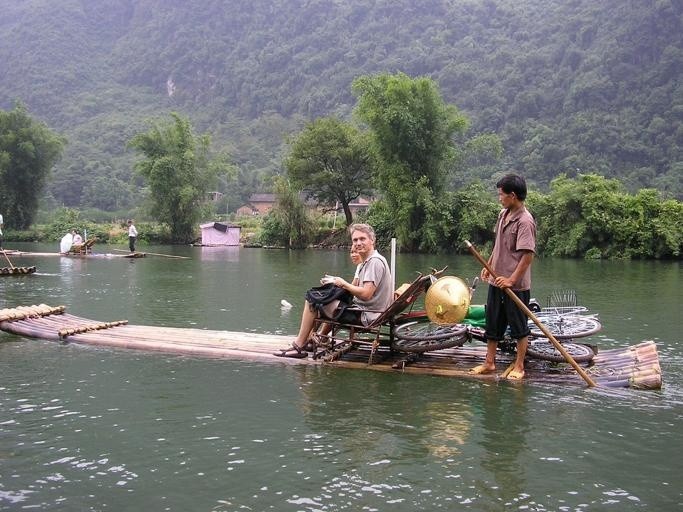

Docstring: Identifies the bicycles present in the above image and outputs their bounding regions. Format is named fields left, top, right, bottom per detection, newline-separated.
left=392, top=297, right=603, bottom=364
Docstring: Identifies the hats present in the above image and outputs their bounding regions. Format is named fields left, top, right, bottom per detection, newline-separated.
left=425, top=276, right=470, bottom=325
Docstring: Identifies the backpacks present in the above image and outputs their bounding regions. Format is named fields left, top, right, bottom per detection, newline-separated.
left=305, top=283, right=353, bottom=320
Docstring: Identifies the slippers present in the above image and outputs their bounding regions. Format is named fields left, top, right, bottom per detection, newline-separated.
left=469, top=366, right=489, bottom=374
left=507, top=369, right=524, bottom=380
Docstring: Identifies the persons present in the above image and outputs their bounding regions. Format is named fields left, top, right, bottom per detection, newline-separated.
left=127, top=221, right=138, bottom=255
left=313, top=243, right=363, bottom=346
left=0, top=213, right=6, bottom=252
left=274, top=223, right=393, bottom=359
left=466, top=174, right=536, bottom=381
left=71, top=229, right=83, bottom=245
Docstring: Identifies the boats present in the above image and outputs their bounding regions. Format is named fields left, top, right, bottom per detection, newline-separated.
left=1, top=298, right=661, bottom=404
left=0, top=266, right=37, bottom=275
left=0, top=247, right=144, bottom=258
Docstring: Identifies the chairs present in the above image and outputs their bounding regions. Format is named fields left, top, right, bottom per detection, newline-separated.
left=313, top=267, right=447, bottom=367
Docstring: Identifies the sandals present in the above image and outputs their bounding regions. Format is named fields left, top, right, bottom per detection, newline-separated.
left=274, top=342, right=308, bottom=357
